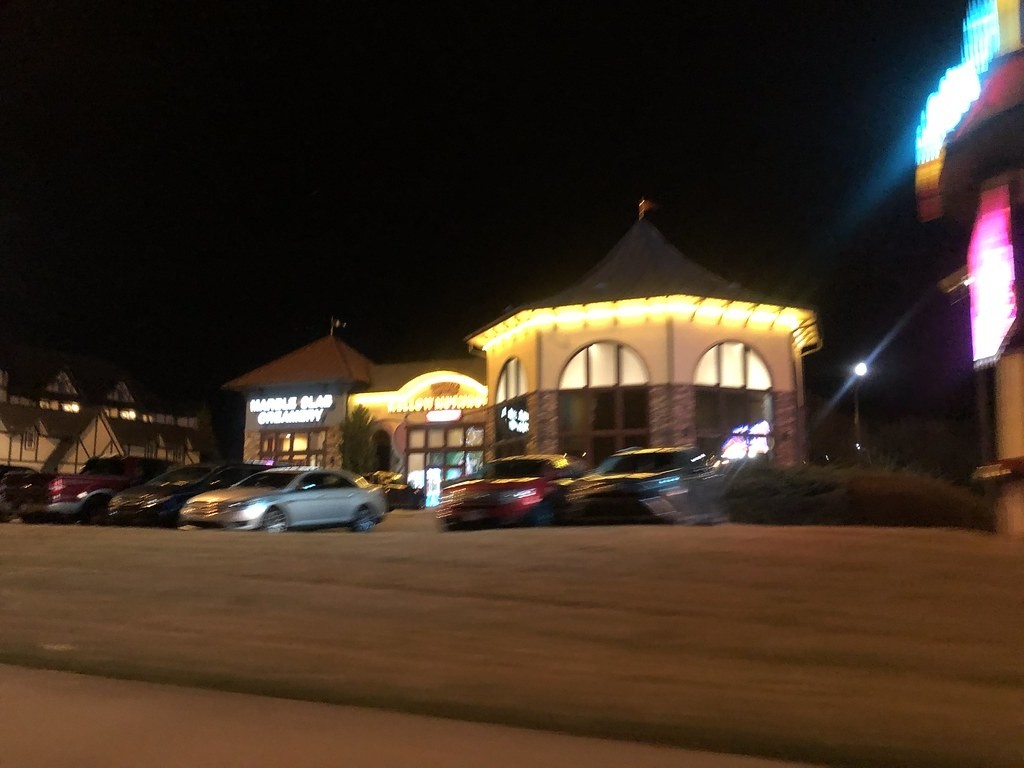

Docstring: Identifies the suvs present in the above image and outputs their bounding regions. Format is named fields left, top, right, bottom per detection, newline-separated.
left=43, top=454, right=183, bottom=527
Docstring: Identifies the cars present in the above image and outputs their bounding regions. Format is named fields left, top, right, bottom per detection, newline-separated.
left=180, top=465, right=389, bottom=536
left=355, top=471, right=426, bottom=514
left=439, top=452, right=590, bottom=530
left=560, top=444, right=731, bottom=526
left=105, top=460, right=275, bottom=529
left=0, top=464, right=51, bottom=524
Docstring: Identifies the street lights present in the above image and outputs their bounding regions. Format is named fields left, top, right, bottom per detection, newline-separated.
left=852, top=361, right=868, bottom=445
